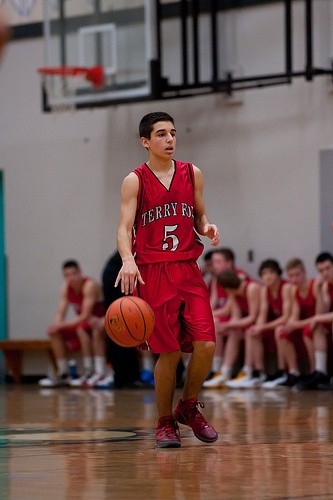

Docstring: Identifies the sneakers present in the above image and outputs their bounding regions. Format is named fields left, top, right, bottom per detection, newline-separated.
left=136, top=369, right=156, bottom=387
left=203, top=372, right=229, bottom=389
left=39, top=368, right=113, bottom=388
left=156, top=416, right=181, bottom=447
left=240, top=368, right=333, bottom=390
left=172, top=398, right=218, bottom=442
left=222, top=372, right=249, bottom=388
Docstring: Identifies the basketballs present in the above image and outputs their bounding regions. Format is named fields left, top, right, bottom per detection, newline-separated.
left=104, top=296, right=155, bottom=348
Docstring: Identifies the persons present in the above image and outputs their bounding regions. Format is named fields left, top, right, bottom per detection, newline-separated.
left=101, top=251, right=142, bottom=390
left=40, top=260, right=115, bottom=388
left=115, top=113, right=221, bottom=447
left=200, top=248, right=333, bottom=391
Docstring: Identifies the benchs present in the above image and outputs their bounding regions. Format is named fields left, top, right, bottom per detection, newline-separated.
left=0, top=338, right=52, bottom=380
